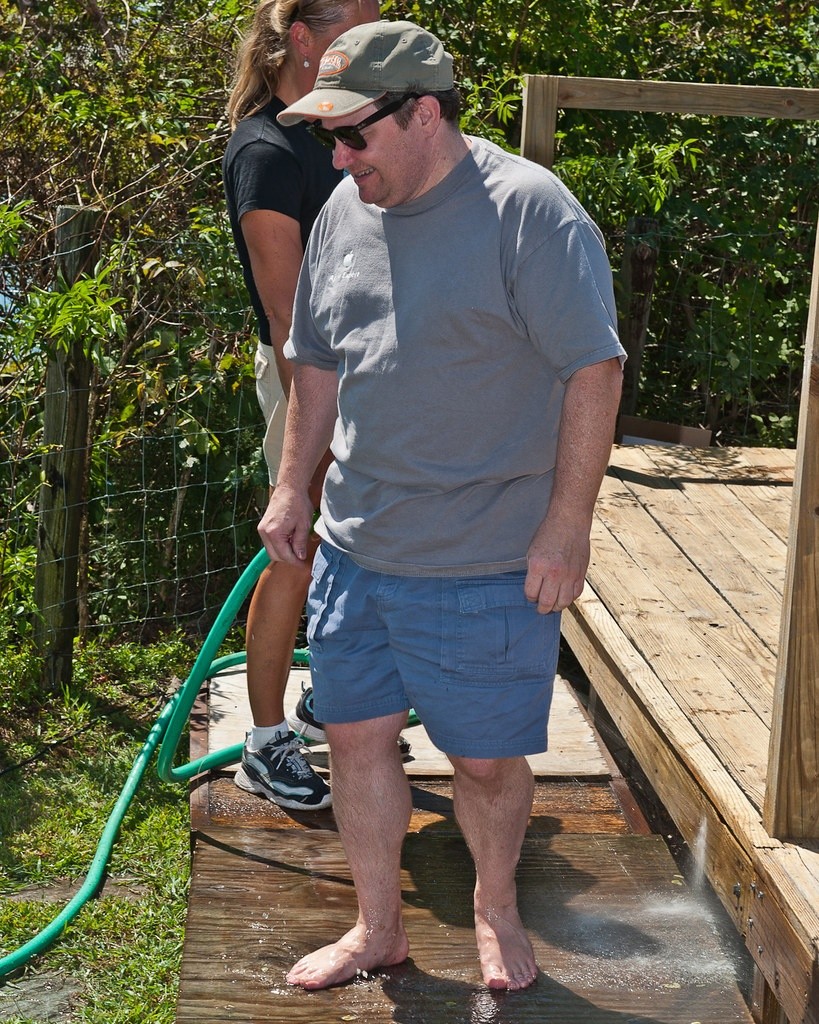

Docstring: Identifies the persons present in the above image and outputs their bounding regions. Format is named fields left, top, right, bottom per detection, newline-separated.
left=258, top=21, right=630, bottom=989
left=222, top=0, right=409, bottom=811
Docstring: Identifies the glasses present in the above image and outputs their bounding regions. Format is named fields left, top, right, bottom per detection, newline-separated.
left=305, top=92, right=420, bottom=151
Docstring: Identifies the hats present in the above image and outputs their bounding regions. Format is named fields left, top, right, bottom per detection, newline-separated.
left=275, top=20, right=455, bottom=128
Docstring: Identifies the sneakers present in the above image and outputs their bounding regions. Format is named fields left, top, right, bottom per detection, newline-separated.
left=289, top=680, right=412, bottom=758
left=233, top=731, right=332, bottom=809
